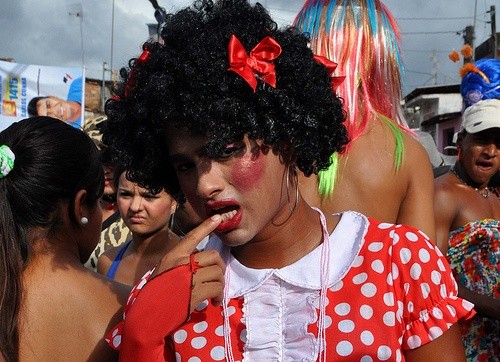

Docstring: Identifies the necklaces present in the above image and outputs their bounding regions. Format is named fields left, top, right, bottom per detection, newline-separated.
left=222, top=206, right=332, bottom=362
left=450, top=168, right=489, bottom=198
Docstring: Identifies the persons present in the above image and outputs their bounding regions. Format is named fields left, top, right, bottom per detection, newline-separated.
left=0, top=114, right=132, bottom=362
left=27, top=75, right=83, bottom=129
left=293, top=1, right=435, bottom=245
left=170, top=191, right=202, bottom=237
left=83, top=150, right=131, bottom=272
left=95, top=169, right=197, bottom=286
left=95, top=0, right=476, bottom=362
left=434, top=98, right=499, bottom=362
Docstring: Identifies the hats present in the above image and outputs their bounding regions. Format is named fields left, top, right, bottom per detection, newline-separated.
left=459, top=98, right=500, bottom=133
